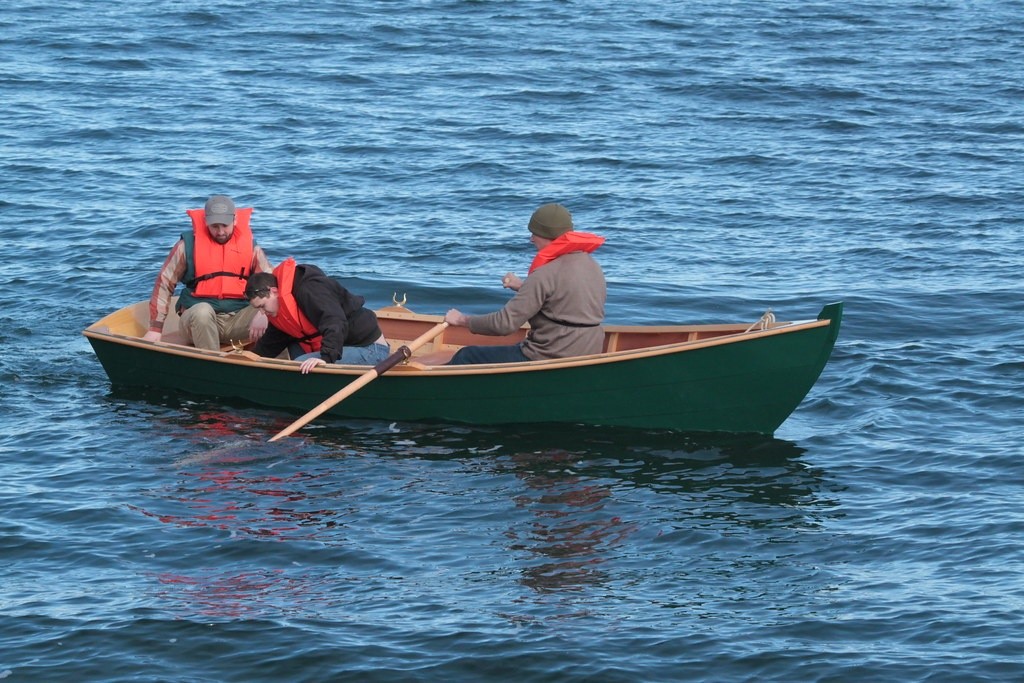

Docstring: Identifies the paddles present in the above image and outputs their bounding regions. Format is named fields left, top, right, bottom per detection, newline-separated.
left=268, top=322, right=449, bottom=441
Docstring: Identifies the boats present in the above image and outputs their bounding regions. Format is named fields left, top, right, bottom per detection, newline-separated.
left=83, top=293, right=846, bottom=439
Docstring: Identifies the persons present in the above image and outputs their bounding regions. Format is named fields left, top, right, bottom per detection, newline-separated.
left=444, top=203, right=606, bottom=366
left=142, top=196, right=277, bottom=352
left=245, top=264, right=390, bottom=374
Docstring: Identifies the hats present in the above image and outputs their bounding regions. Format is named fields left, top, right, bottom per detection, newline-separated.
left=528, top=204, right=573, bottom=239
left=205, top=196, right=235, bottom=226
